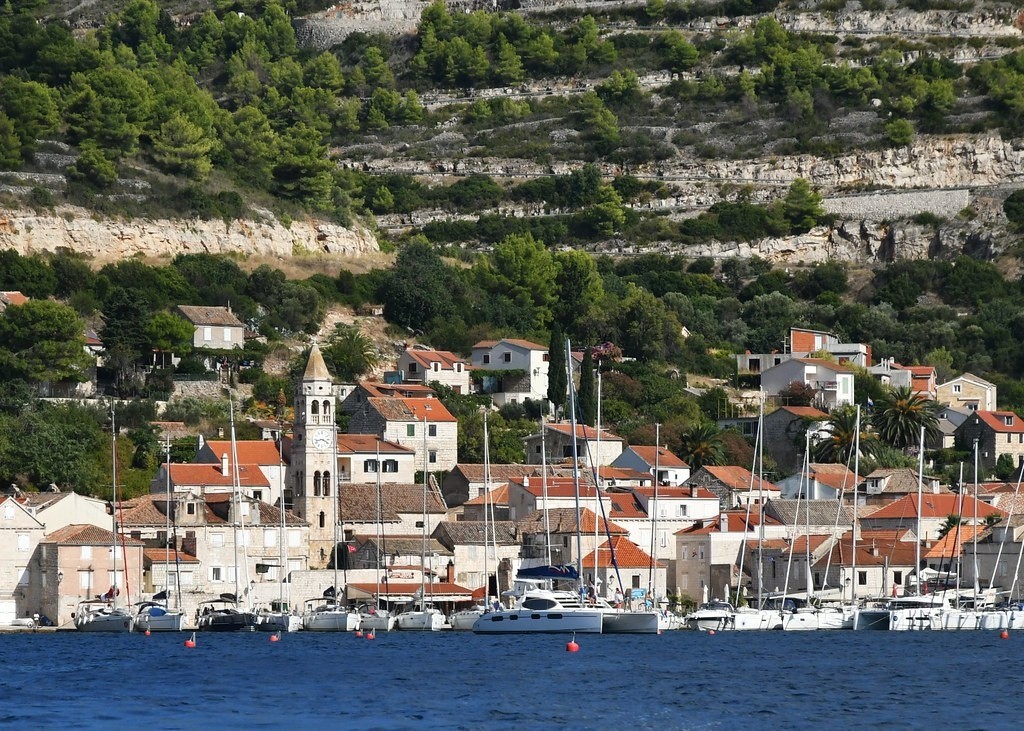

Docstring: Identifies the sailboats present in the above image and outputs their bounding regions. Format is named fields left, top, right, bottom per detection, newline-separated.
left=133, top=431, right=185, bottom=633
left=459, top=339, right=1014, bottom=633
left=74, top=398, right=137, bottom=634
left=399, top=412, right=448, bottom=632
left=309, top=438, right=359, bottom=631
left=193, top=397, right=258, bottom=636
left=354, top=434, right=400, bottom=632
left=259, top=438, right=304, bottom=634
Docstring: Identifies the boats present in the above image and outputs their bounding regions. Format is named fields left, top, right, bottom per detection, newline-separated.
left=467, top=588, right=606, bottom=634
left=10, top=618, right=36, bottom=627
left=38, top=615, right=53, bottom=628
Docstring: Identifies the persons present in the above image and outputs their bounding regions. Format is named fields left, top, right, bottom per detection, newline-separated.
left=585, top=581, right=596, bottom=609
left=614, top=588, right=622, bottom=608
left=645, top=591, right=653, bottom=610
left=33, top=611, right=40, bottom=629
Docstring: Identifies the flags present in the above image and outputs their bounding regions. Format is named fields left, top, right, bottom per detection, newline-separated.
left=348, top=545, right=357, bottom=554
